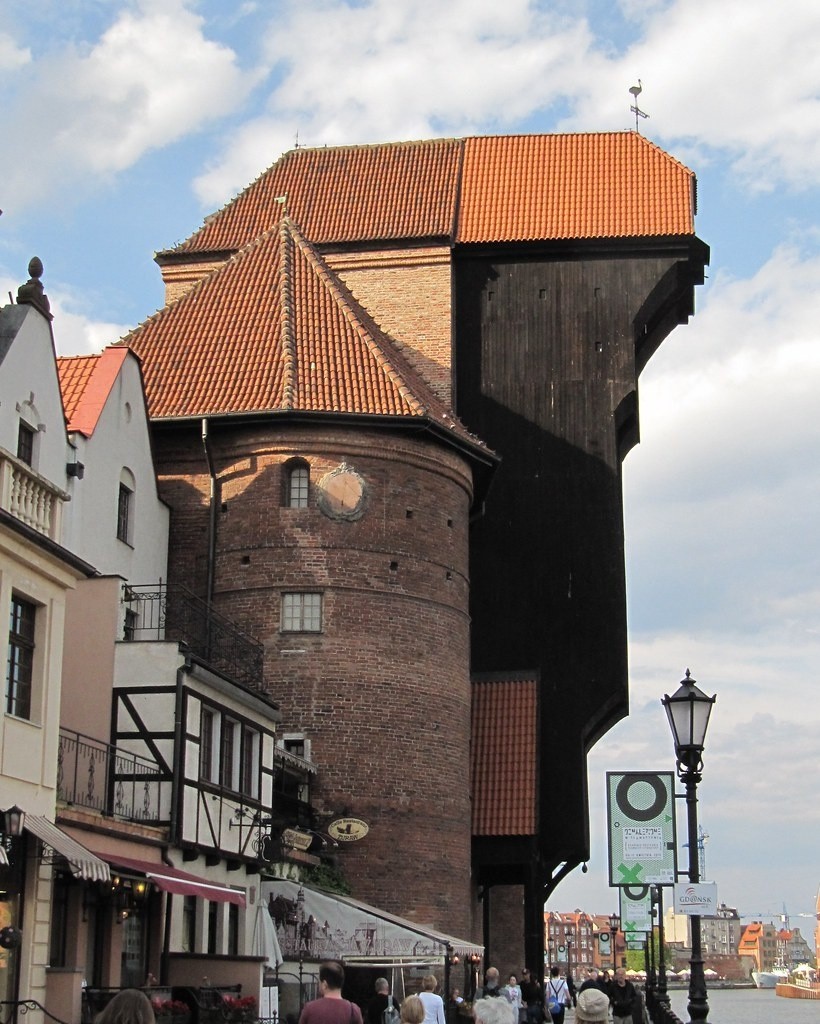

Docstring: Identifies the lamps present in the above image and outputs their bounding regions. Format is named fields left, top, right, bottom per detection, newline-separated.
left=117, top=888, right=132, bottom=925
left=0, top=805, right=25, bottom=846
left=131, top=880, right=151, bottom=902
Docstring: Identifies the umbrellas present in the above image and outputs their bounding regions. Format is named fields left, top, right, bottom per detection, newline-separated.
left=252, top=899, right=283, bottom=972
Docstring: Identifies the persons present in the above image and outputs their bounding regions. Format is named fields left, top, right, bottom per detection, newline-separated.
left=94, top=989, right=156, bottom=1024
left=298, top=960, right=365, bottom=1024
left=373, top=967, right=637, bottom=1024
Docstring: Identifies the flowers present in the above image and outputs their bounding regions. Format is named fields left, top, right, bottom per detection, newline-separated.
left=151, top=996, right=190, bottom=1015
left=219, top=994, right=257, bottom=1011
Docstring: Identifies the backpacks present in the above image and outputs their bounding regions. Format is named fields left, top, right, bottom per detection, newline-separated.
left=546, top=980, right=564, bottom=1014
left=381, top=994, right=401, bottom=1024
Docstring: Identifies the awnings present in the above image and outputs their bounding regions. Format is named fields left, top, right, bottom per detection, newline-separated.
left=21, top=816, right=484, bottom=960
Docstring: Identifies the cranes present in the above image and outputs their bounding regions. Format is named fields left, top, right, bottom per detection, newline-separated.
left=736, top=902, right=816, bottom=929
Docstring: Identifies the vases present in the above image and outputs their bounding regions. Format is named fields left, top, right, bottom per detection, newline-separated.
left=155, top=1014, right=189, bottom=1024
left=224, top=1009, right=254, bottom=1024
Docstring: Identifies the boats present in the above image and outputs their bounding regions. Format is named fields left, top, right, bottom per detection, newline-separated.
left=750, top=966, right=790, bottom=989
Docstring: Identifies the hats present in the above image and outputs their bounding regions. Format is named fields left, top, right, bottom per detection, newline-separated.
left=522, top=968, right=530, bottom=974
left=576, top=988, right=610, bottom=1022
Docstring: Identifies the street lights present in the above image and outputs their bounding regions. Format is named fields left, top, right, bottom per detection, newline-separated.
left=608, top=913, right=621, bottom=975
left=546, top=936, right=556, bottom=972
left=565, top=930, right=574, bottom=981
left=659, top=666, right=718, bottom=1024
